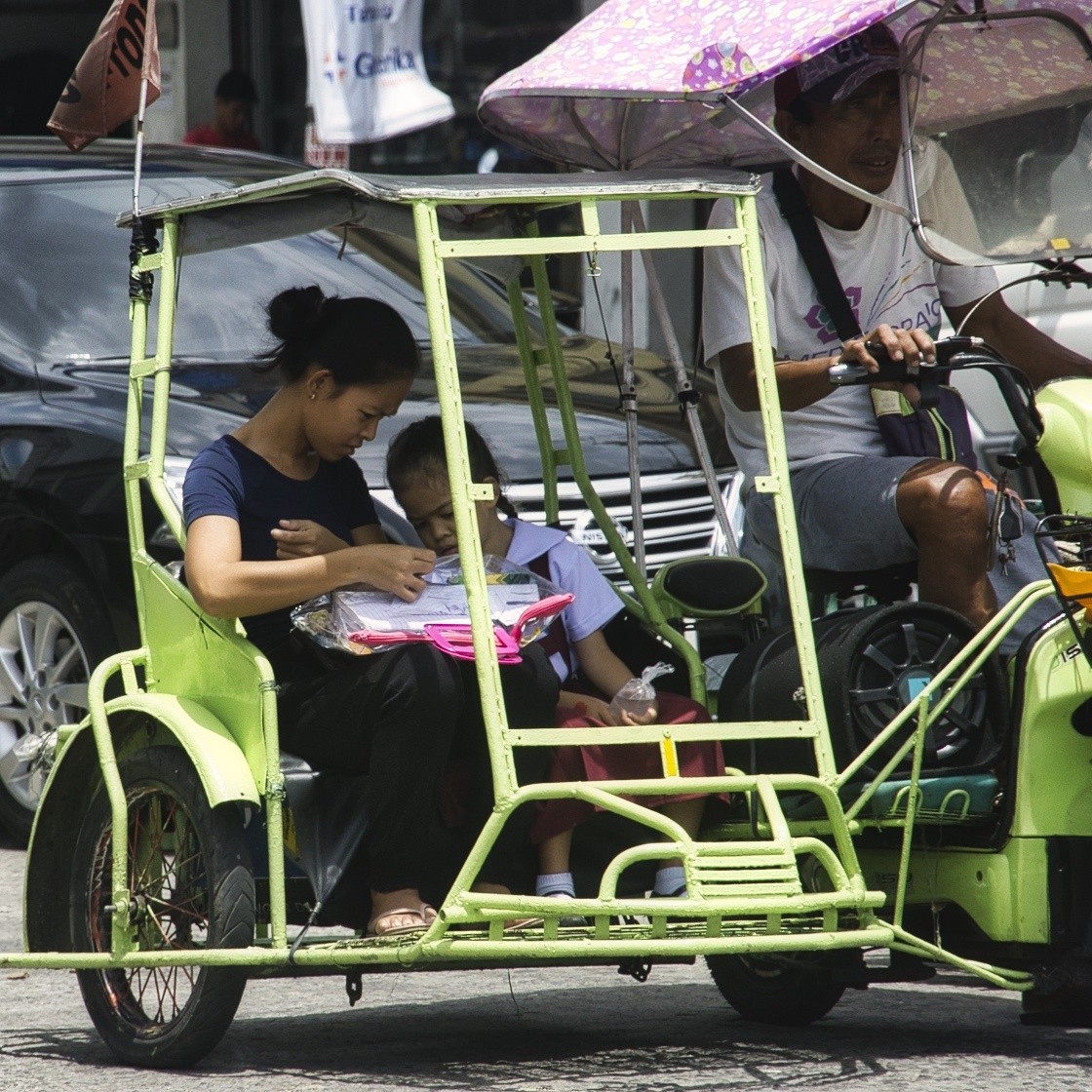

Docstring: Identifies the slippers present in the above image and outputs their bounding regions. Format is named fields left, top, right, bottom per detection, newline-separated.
left=461, top=897, right=544, bottom=931
left=364, top=901, right=441, bottom=937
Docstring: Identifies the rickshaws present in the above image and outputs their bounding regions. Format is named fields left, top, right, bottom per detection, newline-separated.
left=0, top=3, right=1092, bottom=1069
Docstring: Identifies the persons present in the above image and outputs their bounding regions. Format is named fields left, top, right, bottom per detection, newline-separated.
left=181, top=284, right=560, bottom=936
left=184, top=69, right=260, bottom=149
left=702, top=22, right=1092, bottom=655
left=384, top=416, right=731, bottom=927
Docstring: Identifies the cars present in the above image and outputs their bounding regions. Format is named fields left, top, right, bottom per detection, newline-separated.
left=0, top=134, right=757, bottom=850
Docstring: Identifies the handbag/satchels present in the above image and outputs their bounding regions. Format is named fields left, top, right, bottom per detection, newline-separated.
left=870, top=376, right=977, bottom=470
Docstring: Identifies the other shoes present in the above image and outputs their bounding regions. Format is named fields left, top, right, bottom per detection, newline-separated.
left=541, top=892, right=590, bottom=928
left=645, top=882, right=706, bottom=922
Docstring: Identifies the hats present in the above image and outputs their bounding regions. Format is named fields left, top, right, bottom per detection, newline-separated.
left=775, top=21, right=932, bottom=126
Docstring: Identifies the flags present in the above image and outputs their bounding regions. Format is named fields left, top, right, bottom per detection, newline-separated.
left=47, top=0, right=162, bottom=153
